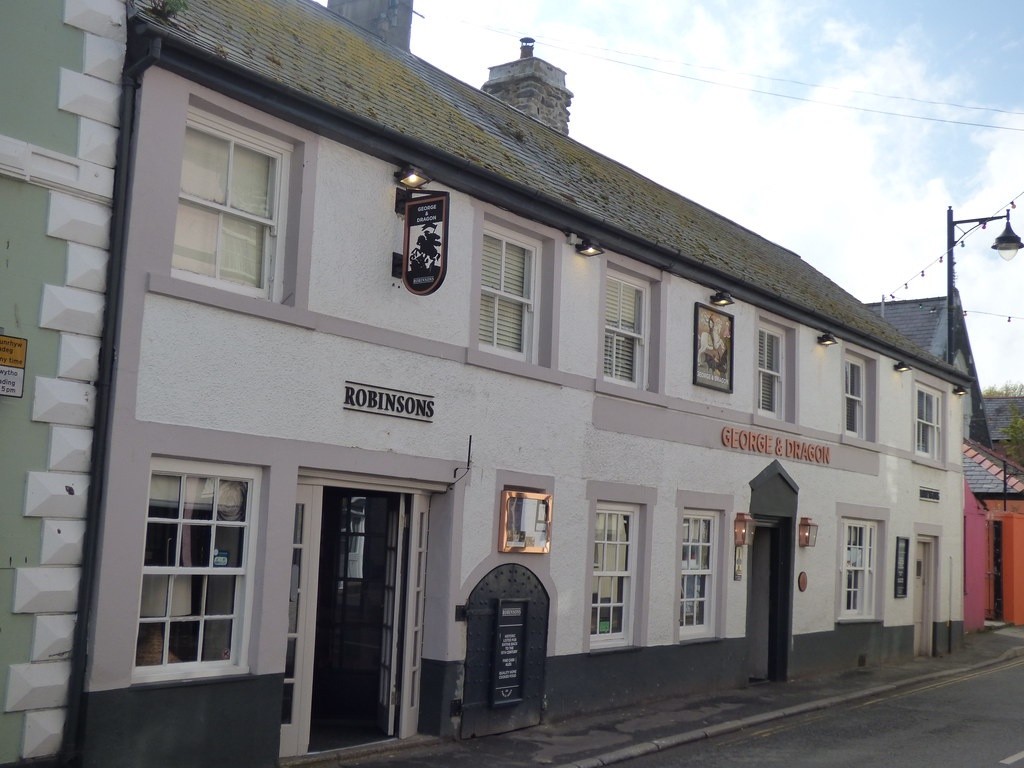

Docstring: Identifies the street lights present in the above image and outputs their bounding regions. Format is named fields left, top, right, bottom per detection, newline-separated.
left=946, top=204, right=1024, bottom=368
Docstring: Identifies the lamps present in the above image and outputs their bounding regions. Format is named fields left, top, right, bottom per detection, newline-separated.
left=395, top=164, right=433, bottom=189
left=894, top=361, right=912, bottom=372
left=575, top=237, right=605, bottom=256
left=953, top=387, right=967, bottom=396
left=711, top=291, right=734, bottom=305
left=799, top=517, right=818, bottom=547
left=734, top=512, right=753, bottom=547
left=817, top=331, right=838, bottom=346
